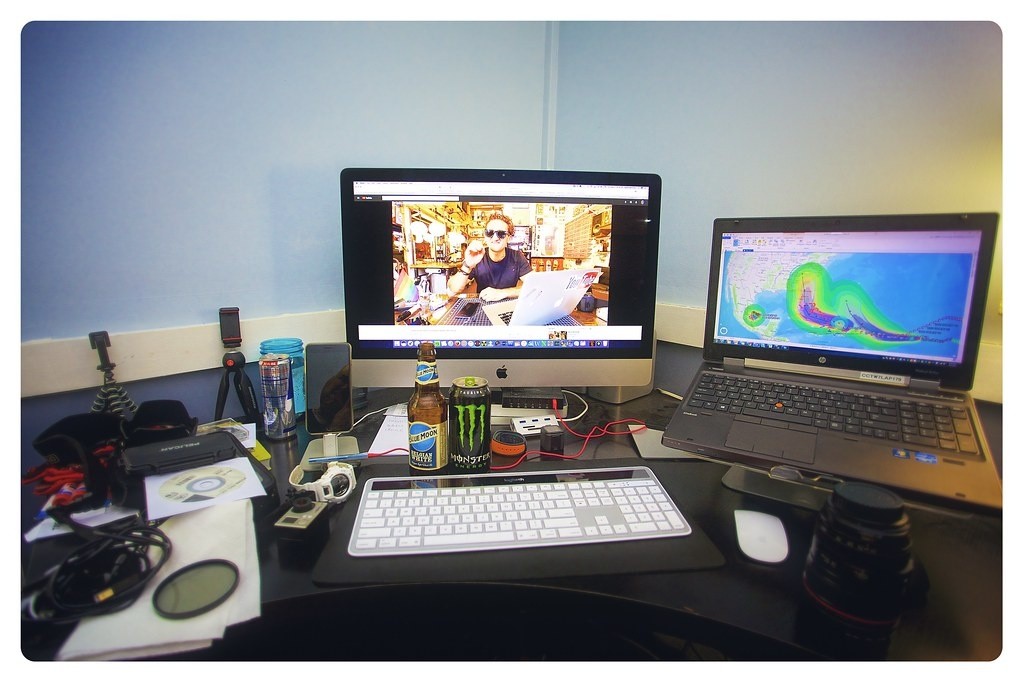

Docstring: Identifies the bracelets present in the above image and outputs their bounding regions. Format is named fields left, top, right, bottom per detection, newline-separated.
left=458, top=268, right=470, bottom=275
left=491, top=430, right=528, bottom=457
left=462, top=259, right=473, bottom=270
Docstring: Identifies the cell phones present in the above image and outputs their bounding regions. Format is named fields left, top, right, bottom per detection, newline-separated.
left=304, top=343, right=354, bottom=436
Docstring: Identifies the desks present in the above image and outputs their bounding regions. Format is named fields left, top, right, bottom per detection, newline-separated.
left=22, top=338, right=1003, bottom=661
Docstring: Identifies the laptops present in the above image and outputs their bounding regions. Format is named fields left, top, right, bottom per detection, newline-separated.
left=662, top=212, right=1003, bottom=520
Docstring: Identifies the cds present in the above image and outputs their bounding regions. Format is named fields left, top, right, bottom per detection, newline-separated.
left=158, top=465, right=246, bottom=503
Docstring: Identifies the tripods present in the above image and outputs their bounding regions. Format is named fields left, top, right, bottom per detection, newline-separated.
left=89, top=330, right=139, bottom=420
left=213, top=306, right=263, bottom=433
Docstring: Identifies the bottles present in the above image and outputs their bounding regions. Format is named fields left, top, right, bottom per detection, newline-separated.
left=802, top=482, right=917, bottom=626
left=407, top=343, right=451, bottom=476
left=259, top=338, right=306, bottom=420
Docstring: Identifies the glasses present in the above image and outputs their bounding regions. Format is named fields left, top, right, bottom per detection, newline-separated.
left=485, top=229, right=510, bottom=239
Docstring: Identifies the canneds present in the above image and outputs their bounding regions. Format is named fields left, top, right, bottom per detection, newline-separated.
left=258, top=353, right=297, bottom=441
left=449, top=376, right=492, bottom=473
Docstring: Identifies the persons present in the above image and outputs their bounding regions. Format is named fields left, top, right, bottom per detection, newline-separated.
left=570, top=259, right=583, bottom=271
left=457, top=243, right=467, bottom=261
left=448, top=213, right=535, bottom=303
left=307, top=363, right=352, bottom=433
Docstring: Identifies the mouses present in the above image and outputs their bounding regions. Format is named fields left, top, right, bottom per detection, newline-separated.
left=732, top=509, right=788, bottom=563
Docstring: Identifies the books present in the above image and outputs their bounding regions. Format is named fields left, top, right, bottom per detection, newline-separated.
left=195, top=417, right=272, bottom=471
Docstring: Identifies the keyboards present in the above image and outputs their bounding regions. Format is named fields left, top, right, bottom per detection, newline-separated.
left=347, top=466, right=693, bottom=557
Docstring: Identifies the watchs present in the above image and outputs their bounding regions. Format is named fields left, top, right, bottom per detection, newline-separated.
left=288, top=461, right=356, bottom=506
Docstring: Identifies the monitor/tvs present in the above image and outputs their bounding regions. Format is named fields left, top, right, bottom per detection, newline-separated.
left=340, top=168, right=662, bottom=425
